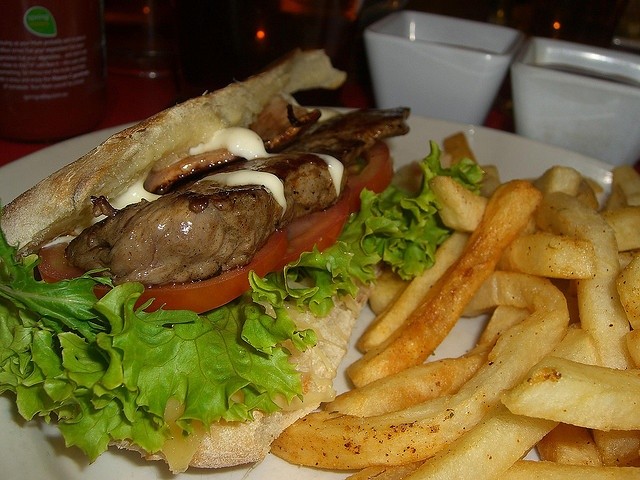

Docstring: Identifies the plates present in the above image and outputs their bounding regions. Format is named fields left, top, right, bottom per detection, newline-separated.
left=1, top=108, right=640, bottom=479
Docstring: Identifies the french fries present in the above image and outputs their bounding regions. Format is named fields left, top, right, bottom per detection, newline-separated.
left=272, top=131, right=640, bottom=480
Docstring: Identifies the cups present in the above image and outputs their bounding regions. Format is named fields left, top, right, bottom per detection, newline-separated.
left=365, top=10, right=519, bottom=127
left=510, top=35, right=640, bottom=167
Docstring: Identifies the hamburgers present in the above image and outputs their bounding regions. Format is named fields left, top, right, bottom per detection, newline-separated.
left=0, top=47, right=483, bottom=474
